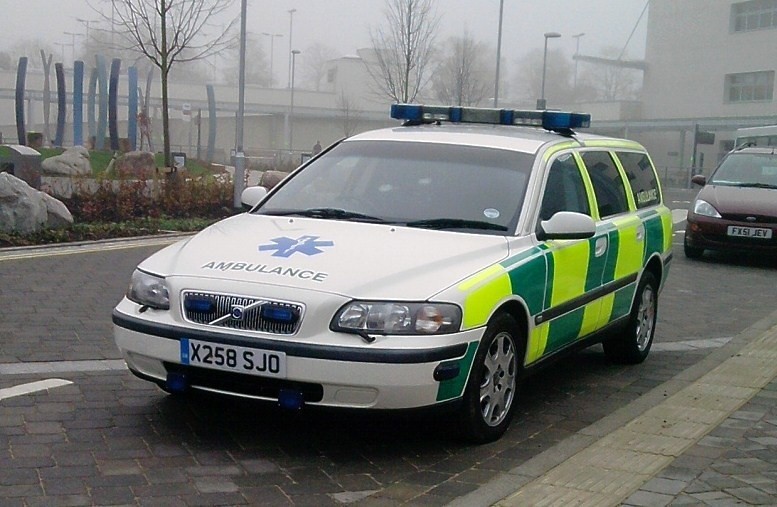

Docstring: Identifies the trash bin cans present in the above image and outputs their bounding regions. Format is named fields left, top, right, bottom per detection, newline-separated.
left=0, top=144, right=42, bottom=192
left=301, top=152, right=312, bottom=164
left=230, top=149, right=236, bottom=166
left=26, top=131, right=43, bottom=148
left=172, top=152, right=186, bottom=172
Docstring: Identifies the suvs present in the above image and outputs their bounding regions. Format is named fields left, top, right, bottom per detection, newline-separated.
left=682, top=141, right=777, bottom=261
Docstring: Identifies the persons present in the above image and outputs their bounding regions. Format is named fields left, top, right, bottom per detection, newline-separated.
left=312, top=140, right=322, bottom=157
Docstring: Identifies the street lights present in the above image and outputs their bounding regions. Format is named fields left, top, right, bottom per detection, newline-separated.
left=287, top=7, right=297, bottom=96
left=571, top=31, right=586, bottom=92
left=63, top=31, right=85, bottom=62
left=540, top=31, right=563, bottom=101
left=53, top=41, right=74, bottom=62
left=288, top=49, right=302, bottom=171
left=78, top=19, right=101, bottom=65
left=261, top=31, right=283, bottom=89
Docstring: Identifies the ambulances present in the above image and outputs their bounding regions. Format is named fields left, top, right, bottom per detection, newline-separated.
left=109, top=103, right=674, bottom=447
